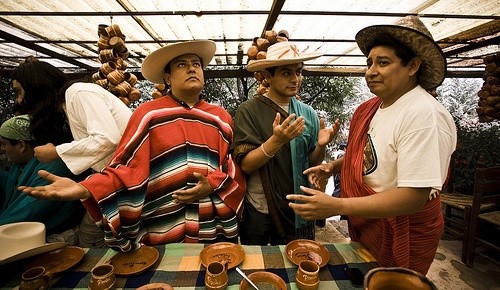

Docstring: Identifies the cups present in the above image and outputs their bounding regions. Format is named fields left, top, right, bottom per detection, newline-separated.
left=246, top=30, right=301, bottom=99
left=295, top=260, right=320, bottom=290
left=88, top=265, right=117, bottom=290
left=475, top=52, right=500, bottom=123
left=204, top=261, right=228, bottom=290
left=91, top=24, right=166, bottom=106
left=97, top=76, right=100, bottom=77
left=21, top=267, right=51, bottom=290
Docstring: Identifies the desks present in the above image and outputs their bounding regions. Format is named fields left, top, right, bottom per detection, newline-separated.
left=437, top=190, right=497, bottom=263
left=45, top=242, right=382, bottom=290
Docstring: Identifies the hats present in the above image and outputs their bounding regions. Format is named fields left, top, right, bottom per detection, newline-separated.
left=245, top=41, right=322, bottom=71
left=1, top=115, right=35, bottom=141
left=142, top=39, right=216, bottom=84
left=355, top=15, right=447, bottom=91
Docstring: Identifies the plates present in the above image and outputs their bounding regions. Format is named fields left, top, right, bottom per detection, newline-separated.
left=199, top=242, right=245, bottom=269
left=26, top=247, right=85, bottom=273
left=285, top=239, right=330, bottom=267
left=108, top=246, right=159, bottom=275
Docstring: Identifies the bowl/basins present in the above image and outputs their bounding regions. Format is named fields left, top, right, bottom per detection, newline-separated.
left=364, top=268, right=438, bottom=290
left=239, top=271, right=287, bottom=290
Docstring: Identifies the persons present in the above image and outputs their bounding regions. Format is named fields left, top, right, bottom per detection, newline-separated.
left=232, top=43, right=341, bottom=247
left=286, top=13, right=457, bottom=276
left=0, top=45, right=246, bottom=246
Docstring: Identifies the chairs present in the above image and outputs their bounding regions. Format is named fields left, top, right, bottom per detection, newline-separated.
left=465, top=165, right=500, bottom=268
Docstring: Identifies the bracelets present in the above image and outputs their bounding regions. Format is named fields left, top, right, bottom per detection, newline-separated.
left=261, top=143, right=275, bottom=158
